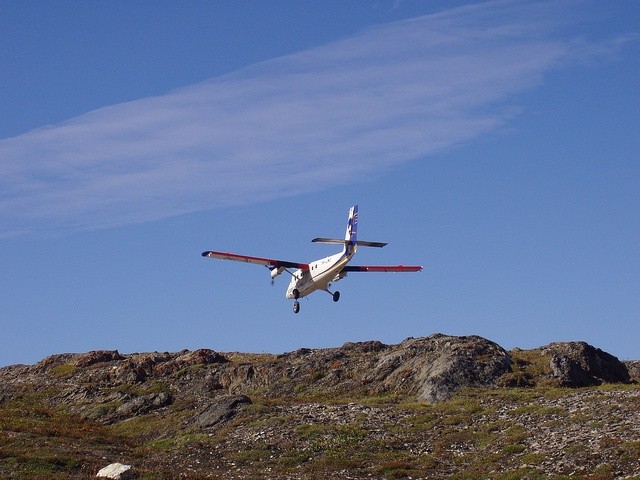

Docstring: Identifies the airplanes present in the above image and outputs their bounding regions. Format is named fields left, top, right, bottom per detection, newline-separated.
left=199, top=203, right=425, bottom=318
